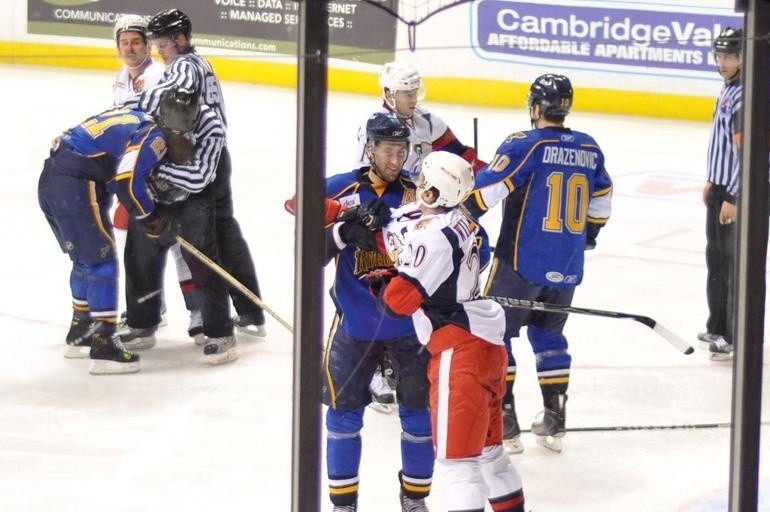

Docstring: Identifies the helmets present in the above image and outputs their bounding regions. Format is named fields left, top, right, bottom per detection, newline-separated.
left=714, top=28, right=745, bottom=54
left=366, top=112, right=411, bottom=142
left=415, top=149, right=476, bottom=209
left=148, top=7, right=192, bottom=38
left=528, top=72, right=574, bottom=116
left=112, top=13, right=148, bottom=37
left=159, top=85, right=200, bottom=133
left=379, top=60, right=421, bottom=91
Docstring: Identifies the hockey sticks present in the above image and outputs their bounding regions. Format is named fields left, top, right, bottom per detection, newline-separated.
left=473, top=294, right=694, bottom=355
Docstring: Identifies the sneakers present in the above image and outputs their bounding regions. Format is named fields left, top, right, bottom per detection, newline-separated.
left=501, top=409, right=521, bottom=441
left=89, top=333, right=140, bottom=363
left=65, top=317, right=94, bottom=346
left=187, top=310, right=203, bottom=337
left=370, top=370, right=395, bottom=405
left=333, top=499, right=358, bottom=512
left=697, top=331, right=720, bottom=342
left=203, top=334, right=237, bottom=355
left=531, top=411, right=566, bottom=437
left=232, top=310, right=264, bottom=327
left=119, top=326, right=154, bottom=343
left=709, top=337, right=734, bottom=354
left=120, top=304, right=167, bottom=318
left=400, top=487, right=430, bottom=512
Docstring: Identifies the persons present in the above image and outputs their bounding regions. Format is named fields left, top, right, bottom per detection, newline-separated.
left=282, top=107, right=435, bottom=511
left=463, top=73, right=616, bottom=439
left=113, top=86, right=237, bottom=354
left=138, top=8, right=266, bottom=326
left=38, top=106, right=196, bottom=364
left=364, top=145, right=524, bottom=511
left=110, top=9, right=208, bottom=336
left=698, top=24, right=746, bottom=355
left=350, top=57, right=490, bottom=403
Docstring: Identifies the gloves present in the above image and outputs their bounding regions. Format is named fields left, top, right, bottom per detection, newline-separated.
left=139, top=213, right=182, bottom=247
left=355, top=198, right=392, bottom=233
